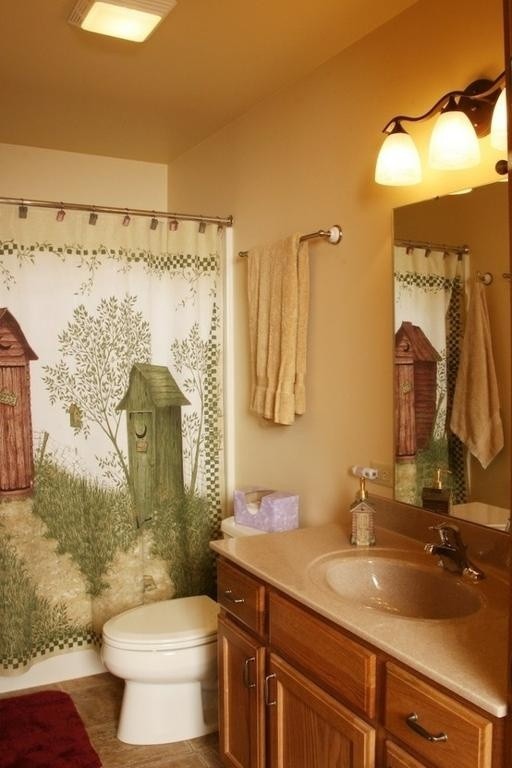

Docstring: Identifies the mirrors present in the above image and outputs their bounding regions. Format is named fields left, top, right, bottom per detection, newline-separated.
left=391, top=179, right=512, bottom=534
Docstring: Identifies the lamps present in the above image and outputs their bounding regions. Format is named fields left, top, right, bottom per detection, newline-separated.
left=65, top=0, right=182, bottom=44
left=374, top=70, right=512, bottom=182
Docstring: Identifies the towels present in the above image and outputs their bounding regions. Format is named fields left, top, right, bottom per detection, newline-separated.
left=245, top=230, right=313, bottom=426
left=450, top=277, right=505, bottom=470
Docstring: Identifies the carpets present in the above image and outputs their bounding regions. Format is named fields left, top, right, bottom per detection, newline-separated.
left=1, top=688, right=103, bottom=767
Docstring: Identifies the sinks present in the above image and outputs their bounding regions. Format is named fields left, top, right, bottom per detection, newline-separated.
left=326, top=557, right=482, bottom=619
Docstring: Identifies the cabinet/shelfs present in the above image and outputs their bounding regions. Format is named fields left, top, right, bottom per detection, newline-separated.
left=378, top=649, right=512, bottom=768
left=209, top=535, right=383, bottom=768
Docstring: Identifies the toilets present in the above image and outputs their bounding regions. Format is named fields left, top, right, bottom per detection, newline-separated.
left=101, top=515, right=268, bottom=745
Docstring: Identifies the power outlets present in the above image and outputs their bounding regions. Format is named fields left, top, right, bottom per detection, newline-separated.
left=367, top=460, right=394, bottom=488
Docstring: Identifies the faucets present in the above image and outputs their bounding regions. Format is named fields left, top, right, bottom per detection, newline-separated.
left=423, top=521, right=485, bottom=582
